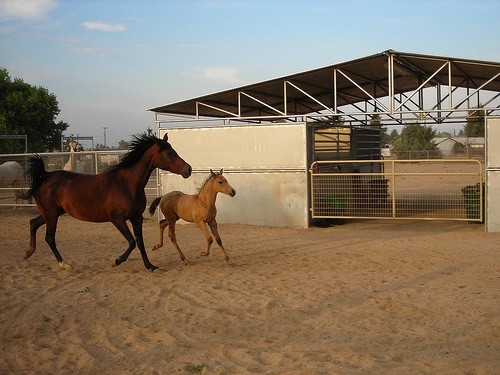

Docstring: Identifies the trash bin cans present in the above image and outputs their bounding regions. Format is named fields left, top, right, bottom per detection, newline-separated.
left=461, top=184, right=485, bottom=225
left=326, top=195, right=344, bottom=222
left=369, top=179, right=389, bottom=208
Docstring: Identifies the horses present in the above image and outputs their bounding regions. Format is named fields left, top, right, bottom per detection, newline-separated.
left=148, top=167, right=237, bottom=266
left=0, top=129, right=192, bottom=273
